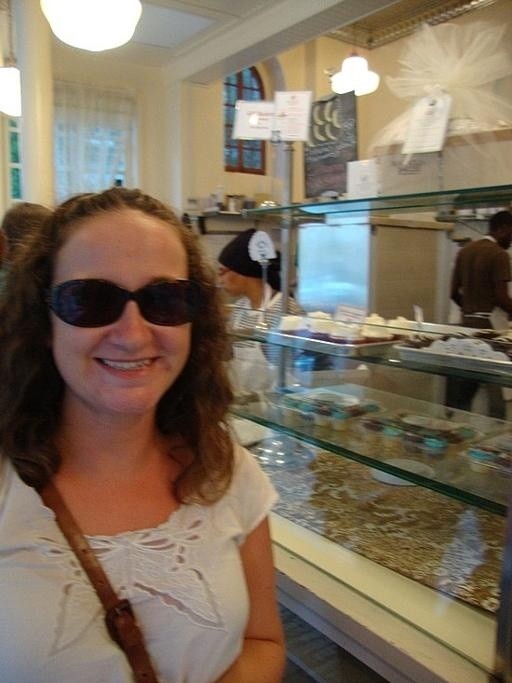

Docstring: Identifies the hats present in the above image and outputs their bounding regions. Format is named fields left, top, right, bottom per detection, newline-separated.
left=218, top=229, right=280, bottom=278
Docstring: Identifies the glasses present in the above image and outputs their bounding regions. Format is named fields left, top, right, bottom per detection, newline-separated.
left=44, top=278, right=206, bottom=329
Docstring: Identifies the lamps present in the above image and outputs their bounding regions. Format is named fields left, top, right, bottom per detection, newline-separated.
left=38, top=0, right=142, bottom=52
left=0, top=1, right=22, bottom=119
left=329, top=22, right=380, bottom=98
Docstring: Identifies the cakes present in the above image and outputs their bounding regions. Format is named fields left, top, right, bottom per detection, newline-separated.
left=278, top=310, right=512, bottom=479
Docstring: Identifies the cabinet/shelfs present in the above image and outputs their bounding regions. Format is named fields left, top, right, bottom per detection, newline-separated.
left=222, top=182, right=512, bottom=516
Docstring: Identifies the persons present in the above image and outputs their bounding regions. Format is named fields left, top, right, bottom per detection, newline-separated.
left=214, top=226, right=309, bottom=395
left=1, top=188, right=288, bottom=682
left=1, top=199, right=56, bottom=269
left=445, top=209, right=512, bottom=422
